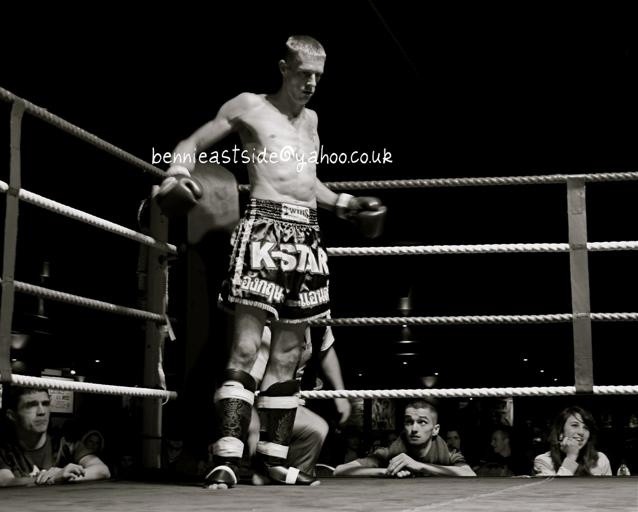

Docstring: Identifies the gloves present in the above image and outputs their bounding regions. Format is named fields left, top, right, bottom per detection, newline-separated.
left=334, top=192, right=388, bottom=239
left=156, top=166, right=202, bottom=216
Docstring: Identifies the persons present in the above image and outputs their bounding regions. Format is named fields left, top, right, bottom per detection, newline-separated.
left=444, top=423, right=462, bottom=451
left=156, top=33, right=390, bottom=489
left=490, top=429, right=532, bottom=475
left=333, top=401, right=478, bottom=479
left=245, top=309, right=354, bottom=486
left=531, top=403, right=614, bottom=477
left=0, top=385, right=113, bottom=488
